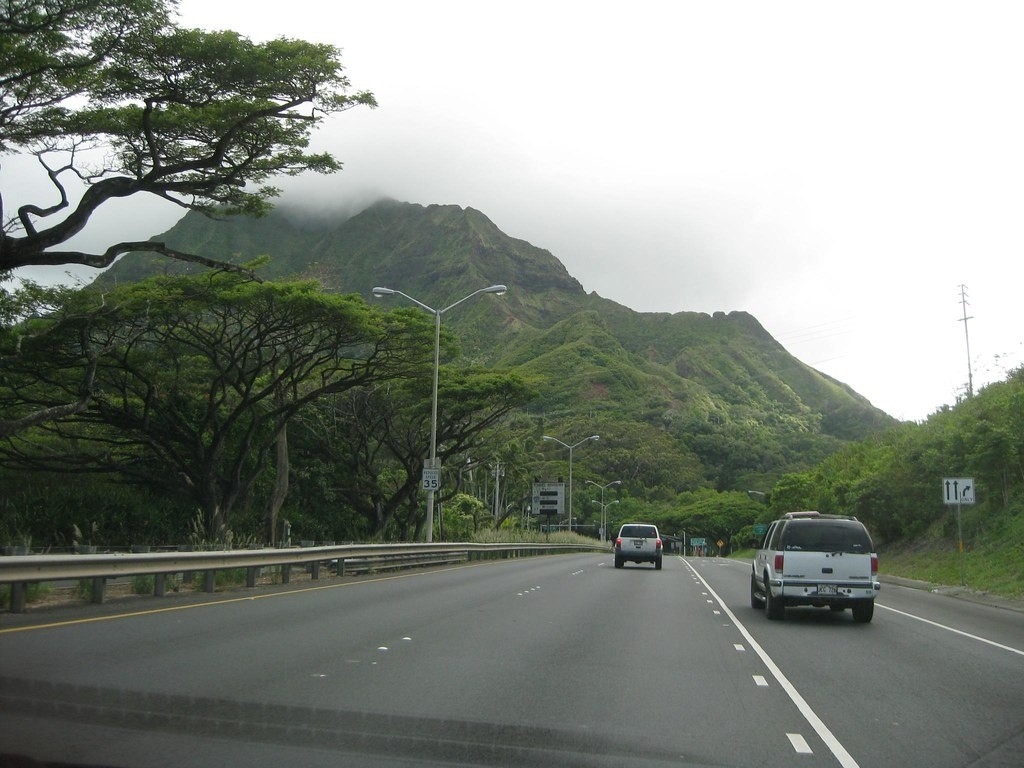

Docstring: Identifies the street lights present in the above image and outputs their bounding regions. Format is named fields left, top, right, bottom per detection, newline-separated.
left=542, top=433, right=599, bottom=531
left=372, top=283, right=507, bottom=543
left=586, top=480, right=622, bottom=541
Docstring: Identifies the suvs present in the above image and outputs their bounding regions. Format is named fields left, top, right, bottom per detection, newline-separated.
left=749, top=509, right=883, bottom=626
left=613, top=522, right=664, bottom=570
